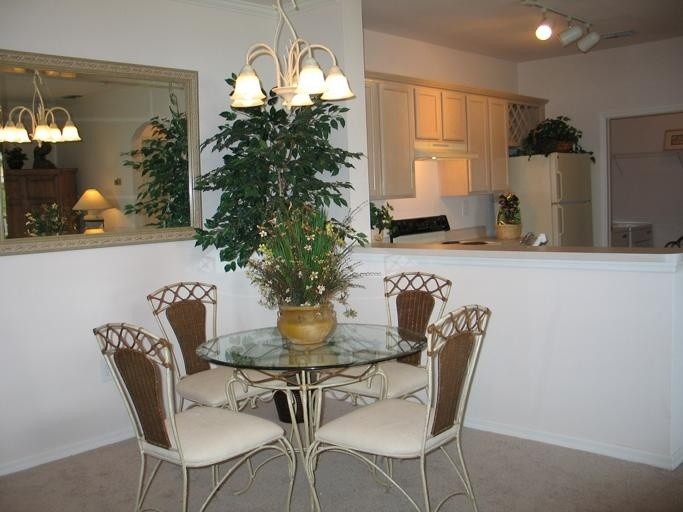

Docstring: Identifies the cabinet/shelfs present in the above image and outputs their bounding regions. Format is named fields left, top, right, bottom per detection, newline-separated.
left=363, top=69, right=510, bottom=202
left=5, top=169, right=77, bottom=239
left=610, top=219, right=653, bottom=248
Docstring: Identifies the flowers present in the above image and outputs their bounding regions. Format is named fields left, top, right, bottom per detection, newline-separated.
left=246, top=201, right=369, bottom=317
left=24, top=202, right=67, bottom=237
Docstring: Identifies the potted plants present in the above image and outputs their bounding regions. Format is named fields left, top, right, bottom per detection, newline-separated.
left=522, top=115, right=596, bottom=165
left=495, top=192, right=522, bottom=241
left=193, top=71, right=369, bottom=424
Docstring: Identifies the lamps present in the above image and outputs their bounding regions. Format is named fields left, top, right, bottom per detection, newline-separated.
left=229, top=1, right=357, bottom=109
left=1, top=66, right=81, bottom=144
left=522, top=2, right=601, bottom=53
left=73, top=188, right=109, bottom=222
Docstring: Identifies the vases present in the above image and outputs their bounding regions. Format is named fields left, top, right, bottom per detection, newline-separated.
left=277, top=305, right=336, bottom=348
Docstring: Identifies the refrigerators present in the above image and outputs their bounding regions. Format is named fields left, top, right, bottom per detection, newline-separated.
left=508, top=152, right=594, bottom=247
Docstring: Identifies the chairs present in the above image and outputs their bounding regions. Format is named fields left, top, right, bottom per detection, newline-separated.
left=317, top=271, right=454, bottom=490
left=92, top=321, right=299, bottom=512
left=146, top=281, right=298, bottom=493
left=301, top=305, right=502, bottom=511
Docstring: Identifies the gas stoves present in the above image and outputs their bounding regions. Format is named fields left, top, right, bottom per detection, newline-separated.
left=434, top=240, right=497, bottom=245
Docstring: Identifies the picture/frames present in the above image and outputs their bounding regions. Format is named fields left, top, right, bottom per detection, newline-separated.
left=663, top=128, right=683, bottom=151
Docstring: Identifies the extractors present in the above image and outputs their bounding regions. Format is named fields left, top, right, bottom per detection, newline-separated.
left=416, top=147, right=479, bottom=162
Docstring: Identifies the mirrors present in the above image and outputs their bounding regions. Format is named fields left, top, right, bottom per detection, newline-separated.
left=1, top=48, right=200, bottom=256
